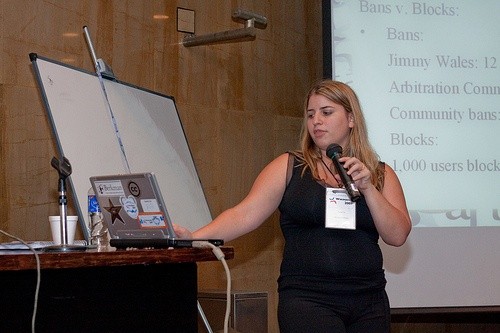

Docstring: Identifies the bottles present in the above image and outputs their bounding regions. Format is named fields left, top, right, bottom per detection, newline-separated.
left=87, top=187, right=108, bottom=248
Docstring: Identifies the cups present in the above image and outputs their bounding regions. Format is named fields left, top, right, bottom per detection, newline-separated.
left=48, top=215, right=78, bottom=245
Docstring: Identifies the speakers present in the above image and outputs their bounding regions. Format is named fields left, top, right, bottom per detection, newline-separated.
left=197, top=289, right=268, bottom=333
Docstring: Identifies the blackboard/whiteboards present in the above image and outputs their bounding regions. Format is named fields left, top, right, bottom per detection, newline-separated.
left=27, top=52, right=222, bottom=243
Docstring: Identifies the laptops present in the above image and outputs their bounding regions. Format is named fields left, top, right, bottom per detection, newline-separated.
left=90, top=172, right=224, bottom=248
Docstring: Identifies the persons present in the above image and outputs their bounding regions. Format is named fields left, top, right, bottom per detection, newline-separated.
left=173, top=80, right=412, bottom=333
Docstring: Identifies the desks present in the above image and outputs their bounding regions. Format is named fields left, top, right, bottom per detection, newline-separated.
left=0, top=247, right=235, bottom=333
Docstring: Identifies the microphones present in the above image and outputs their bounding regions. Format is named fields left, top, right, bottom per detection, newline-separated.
left=326, top=142, right=361, bottom=203
